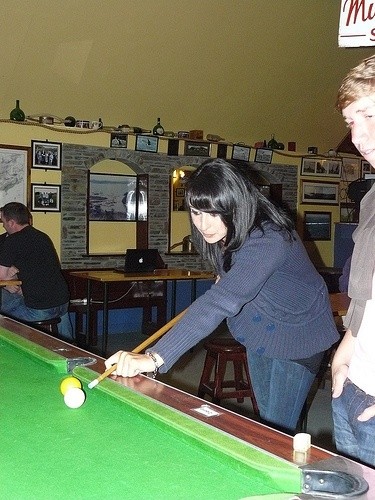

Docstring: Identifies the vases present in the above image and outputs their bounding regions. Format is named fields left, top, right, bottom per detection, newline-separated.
left=153, top=118, right=164, bottom=135
left=10, top=100, right=25, bottom=121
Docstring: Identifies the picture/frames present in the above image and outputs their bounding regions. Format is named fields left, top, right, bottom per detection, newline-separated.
left=31, top=140, right=62, bottom=170
left=0, top=143, right=31, bottom=223
left=176, top=188, right=185, bottom=197
left=299, top=157, right=375, bottom=241
left=110, top=133, right=273, bottom=164
left=31, top=183, right=61, bottom=212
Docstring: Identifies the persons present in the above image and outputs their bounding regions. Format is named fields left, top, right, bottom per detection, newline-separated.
left=331, top=53, right=375, bottom=471
left=0, top=202, right=70, bottom=323
left=35, top=148, right=57, bottom=165
left=148, top=139, right=150, bottom=145
left=104, top=159, right=339, bottom=436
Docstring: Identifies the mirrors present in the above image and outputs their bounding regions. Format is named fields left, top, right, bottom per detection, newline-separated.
left=86, top=160, right=148, bottom=257
left=168, top=165, right=200, bottom=255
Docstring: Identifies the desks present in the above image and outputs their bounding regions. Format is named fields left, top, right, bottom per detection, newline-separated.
left=0, top=314, right=375, bottom=500
left=317, top=293, right=351, bottom=390
left=69, top=269, right=214, bottom=353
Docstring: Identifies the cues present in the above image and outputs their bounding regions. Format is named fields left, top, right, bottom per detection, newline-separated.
left=88, top=304, right=190, bottom=389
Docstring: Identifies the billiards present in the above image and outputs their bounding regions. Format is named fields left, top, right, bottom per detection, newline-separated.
left=64, top=388, right=86, bottom=409
left=61, top=377, right=81, bottom=396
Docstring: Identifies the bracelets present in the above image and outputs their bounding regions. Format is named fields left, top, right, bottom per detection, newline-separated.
left=147, top=351, right=157, bottom=379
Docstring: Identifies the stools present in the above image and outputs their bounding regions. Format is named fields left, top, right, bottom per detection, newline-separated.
left=197, top=338, right=259, bottom=415
left=22, top=317, right=61, bottom=339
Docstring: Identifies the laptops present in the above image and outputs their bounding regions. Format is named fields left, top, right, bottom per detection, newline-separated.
left=112, top=248, right=158, bottom=274
